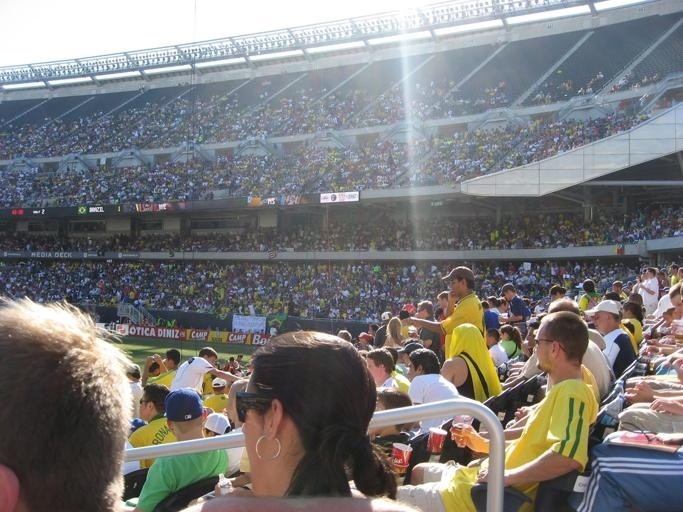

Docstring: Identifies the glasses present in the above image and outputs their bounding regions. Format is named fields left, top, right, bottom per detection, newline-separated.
left=140, top=399, right=156, bottom=404
left=236, top=387, right=269, bottom=422
left=533, top=339, right=567, bottom=352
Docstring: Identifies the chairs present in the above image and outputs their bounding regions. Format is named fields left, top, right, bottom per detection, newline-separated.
left=110, top=323, right=662, bottom=510
left=0, top=11, right=683, bottom=139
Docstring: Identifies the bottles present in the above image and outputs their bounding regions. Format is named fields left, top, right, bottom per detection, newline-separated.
left=215, top=473, right=235, bottom=498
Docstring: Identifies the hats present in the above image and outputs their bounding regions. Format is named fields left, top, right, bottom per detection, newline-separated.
left=204, top=413, right=232, bottom=435
left=211, top=377, right=225, bottom=388
left=128, top=364, right=140, bottom=374
left=584, top=300, right=619, bottom=317
left=358, top=332, right=369, bottom=340
left=441, top=267, right=475, bottom=282
left=381, top=312, right=391, bottom=321
left=499, top=284, right=514, bottom=296
left=165, top=389, right=203, bottom=421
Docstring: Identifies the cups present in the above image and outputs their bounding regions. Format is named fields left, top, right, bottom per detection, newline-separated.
left=391, top=443, right=413, bottom=467
left=449, top=415, right=473, bottom=437
left=425, top=426, right=447, bottom=455
